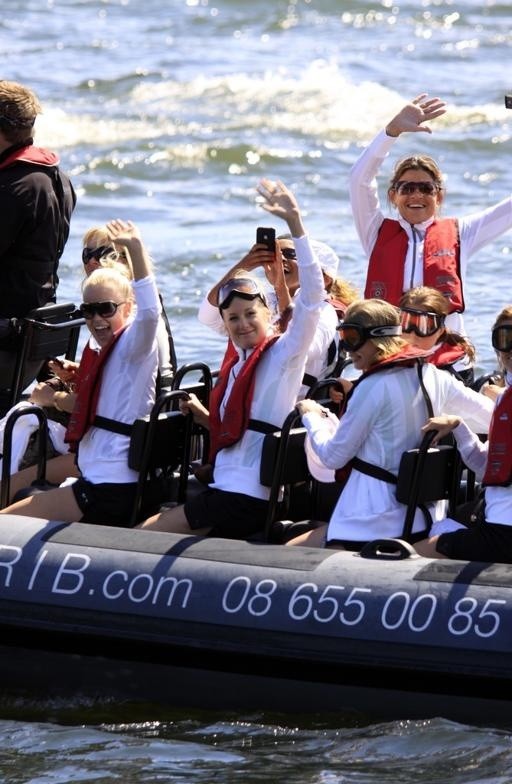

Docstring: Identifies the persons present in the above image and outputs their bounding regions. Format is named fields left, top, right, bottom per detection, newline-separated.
left=1, top=80, right=78, bottom=421
left=133, top=179, right=327, bottom=537
left=346, top=93, right=512, bottom=342
left=331, top=286, right=474, bottom=404
left=1, top=225, right=175, bottom=503
left=420, top=306, right=511, bottom=564
left=207, top=234, right=339, bottom=407
left=285, top=298, right=495, bottom=553
left=306, top=239, right=358, bottom=319
left=1, top=219, right=161, bottom=529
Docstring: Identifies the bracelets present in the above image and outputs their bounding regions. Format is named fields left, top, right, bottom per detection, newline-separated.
left=53, top=391, right=65, bottom=411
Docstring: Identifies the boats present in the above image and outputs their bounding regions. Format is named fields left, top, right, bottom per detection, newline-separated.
left=0, top=513, right=512, bottom=683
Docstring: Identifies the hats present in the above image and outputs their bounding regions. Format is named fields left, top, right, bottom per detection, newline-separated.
left=309, top=239, right=339, bottom=286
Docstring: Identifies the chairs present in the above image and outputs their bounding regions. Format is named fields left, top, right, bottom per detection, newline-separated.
left=261, top=408, right=320, bottom=546
left=306, top=380, right=346, bottom=420
left=471, top=372, right=497, bottom=443
left=333, top=358, right=353, bottom=378
left=2, top=303, right=86, bottom=404
left=0, top=408, right=48, bottom=516
left=170, top=361, right=213, bottom=467
left=127, top=390, right=195, bottom=529
left=396, top=425, right=458, bottom=550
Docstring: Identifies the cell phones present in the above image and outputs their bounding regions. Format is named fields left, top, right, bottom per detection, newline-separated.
left=256, top=227, right=276, bottom=264
left=53, top=356, right=63, bottom=370
left=504, top=96, right=512, bottom=110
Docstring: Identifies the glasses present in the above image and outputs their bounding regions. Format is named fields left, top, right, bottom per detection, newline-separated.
left=216, top=278, right=269, bottom=320
left=80, top=300, right=127, bottom=320
left=81, top=245, right=126, bottom=265
left=399, top=306, right=447, bottom=338
left=394, top=180, right=440, bottom=195
left=491, top=324, right=512, bottom=353
left=336, top=322, right=402, bottom=353
left=280, top=248, right=297, bottom=260
